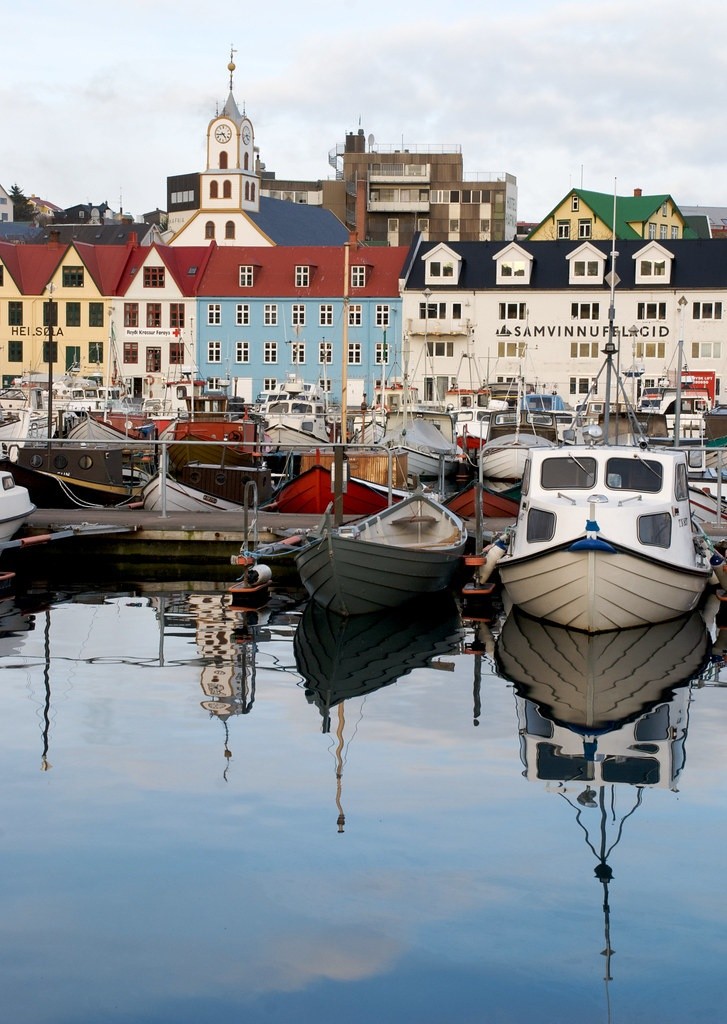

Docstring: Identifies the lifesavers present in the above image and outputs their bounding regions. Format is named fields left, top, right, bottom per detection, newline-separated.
left=144, top=375, right=155, bottom=386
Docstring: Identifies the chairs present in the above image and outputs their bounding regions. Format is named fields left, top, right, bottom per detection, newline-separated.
left=588, top=472, right=622, bottom=489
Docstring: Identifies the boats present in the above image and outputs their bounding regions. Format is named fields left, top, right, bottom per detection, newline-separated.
left=0, top=174, right=727, bottom=635
left=289, top=590, right=466, bottom=735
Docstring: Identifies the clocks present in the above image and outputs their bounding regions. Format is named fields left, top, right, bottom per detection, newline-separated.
left=242, top=126, right=251, bottom=145
left=215, top=124, right=232, bottom=144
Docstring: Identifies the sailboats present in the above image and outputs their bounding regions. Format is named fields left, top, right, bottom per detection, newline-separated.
left=489, top=611, right=716, bottom=987
